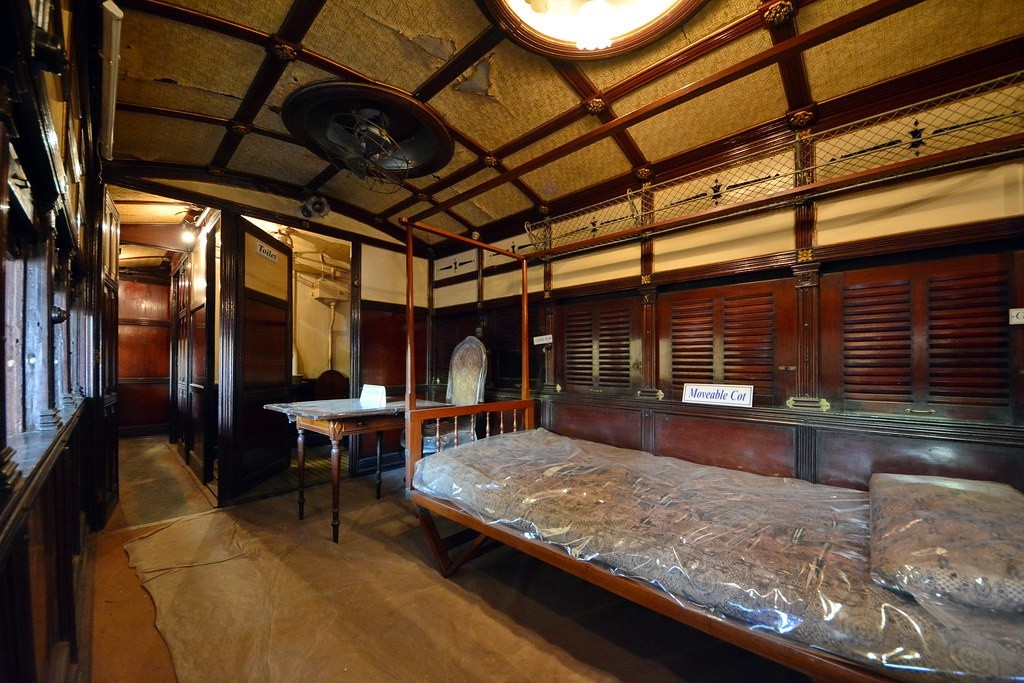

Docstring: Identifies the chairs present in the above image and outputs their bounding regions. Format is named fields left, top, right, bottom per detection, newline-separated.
left=316, top=369, right=347, bottom=447
left=400, top=336, right=488, bottom=457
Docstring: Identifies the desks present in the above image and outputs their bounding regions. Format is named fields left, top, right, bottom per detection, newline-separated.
left=262, top=396, right=456, bottom=543
left=292, top=379, right=350, bottom=446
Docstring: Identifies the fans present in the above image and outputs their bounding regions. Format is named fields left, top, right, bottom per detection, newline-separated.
left=321, top=112, right=409, bottom=193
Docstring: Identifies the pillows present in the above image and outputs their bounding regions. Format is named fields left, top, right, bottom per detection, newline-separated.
left=869, top=472, right=1024, bottom=613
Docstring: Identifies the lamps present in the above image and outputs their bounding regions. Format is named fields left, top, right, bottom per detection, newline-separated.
left=475, top=0, right=711, bottom=63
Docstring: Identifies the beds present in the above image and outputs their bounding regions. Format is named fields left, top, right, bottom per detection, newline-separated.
left=398, top=0, right=1024, bottom=683
